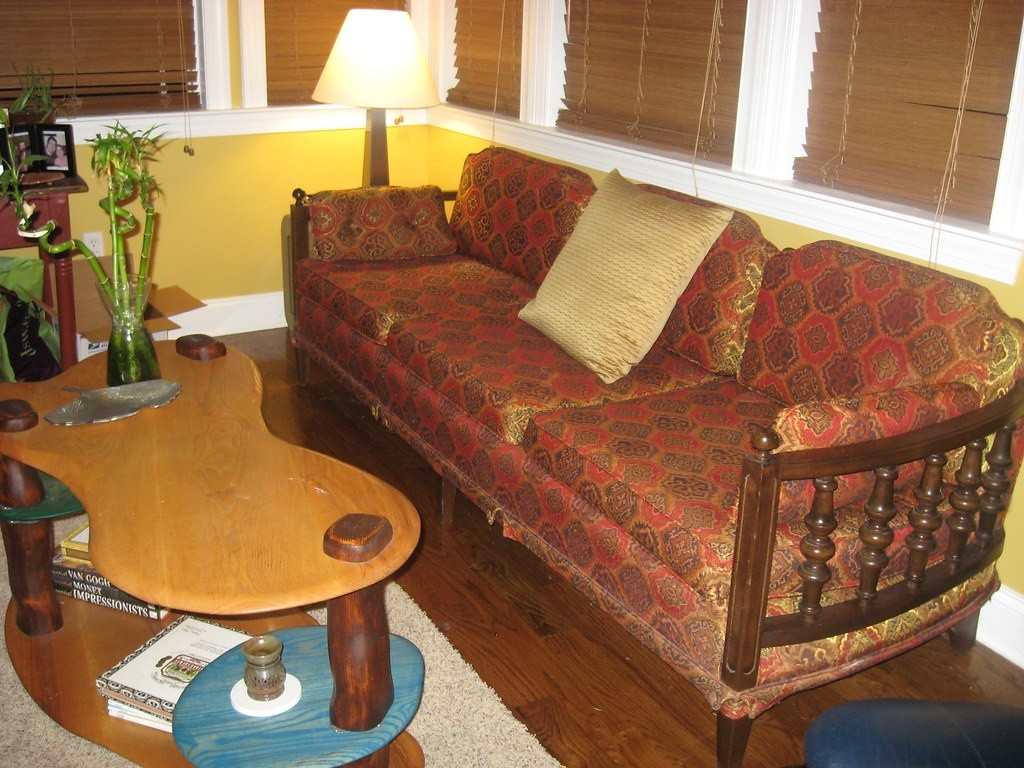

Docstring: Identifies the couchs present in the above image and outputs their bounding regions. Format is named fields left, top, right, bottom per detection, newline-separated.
left=280, top=142, right=1024, bottom=768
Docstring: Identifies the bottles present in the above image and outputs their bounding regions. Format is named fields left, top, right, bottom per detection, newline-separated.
left=243, top=635, right=285, bottom=701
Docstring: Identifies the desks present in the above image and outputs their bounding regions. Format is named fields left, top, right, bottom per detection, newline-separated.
left=0, top=167, right=89, bottom=370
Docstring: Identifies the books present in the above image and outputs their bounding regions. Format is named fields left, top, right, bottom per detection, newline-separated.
left=52, top=521, right=172, bottom=621
left=96, top=613, right=256, bottom=733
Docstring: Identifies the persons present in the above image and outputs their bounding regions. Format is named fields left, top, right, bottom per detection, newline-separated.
left=45, top=137, right=68, bottom=167
left=17, top=141, right=30, bottom=161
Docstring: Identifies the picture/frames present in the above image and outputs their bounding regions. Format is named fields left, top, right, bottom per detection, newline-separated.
left=35, top=122, right=78, bottom=177
left=0, top=123, right=40, bottom=175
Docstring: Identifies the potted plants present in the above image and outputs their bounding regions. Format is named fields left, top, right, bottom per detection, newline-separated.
left=0, top=64, right=165, bottom=387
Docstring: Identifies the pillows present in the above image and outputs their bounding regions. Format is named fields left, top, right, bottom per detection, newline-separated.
left=519, top=169, right=734, bottom=387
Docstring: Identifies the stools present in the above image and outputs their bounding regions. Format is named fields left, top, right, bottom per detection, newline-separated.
left=801, top=699, right=1024, bottom=768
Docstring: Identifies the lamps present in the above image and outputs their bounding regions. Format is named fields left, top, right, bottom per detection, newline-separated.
left=312, top=7, right=441, bottom=187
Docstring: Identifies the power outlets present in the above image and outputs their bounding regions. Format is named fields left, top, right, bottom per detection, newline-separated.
left=83, top=231, right=105, bottom=258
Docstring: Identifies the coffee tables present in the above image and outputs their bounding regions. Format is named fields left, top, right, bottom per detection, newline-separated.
left=0, top=333, right=426, bottom=768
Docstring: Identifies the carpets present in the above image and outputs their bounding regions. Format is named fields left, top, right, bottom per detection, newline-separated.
left=0, top=510, right=564, bottom=768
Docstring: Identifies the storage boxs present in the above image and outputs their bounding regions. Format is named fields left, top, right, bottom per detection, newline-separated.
left=16, top=253, right=207, bottom=363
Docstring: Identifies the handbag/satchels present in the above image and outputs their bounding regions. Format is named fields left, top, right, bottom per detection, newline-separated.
left=0, top=256, right=62, bottom=384
